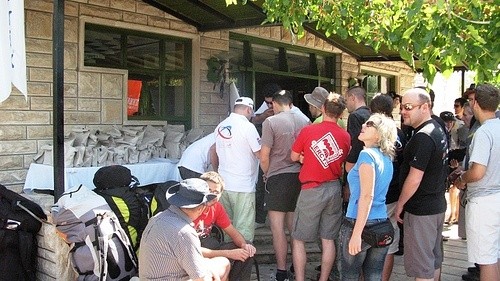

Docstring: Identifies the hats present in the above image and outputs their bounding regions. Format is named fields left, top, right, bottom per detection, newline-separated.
left=234, top=97, right=256, bottom=118
left=464, top=84, right=476, bottom=98
left=440, top=111, right=456, bottom=122
left=166, top=178, right=217, bottom=209
left=304, top=87, right=329, bottom=111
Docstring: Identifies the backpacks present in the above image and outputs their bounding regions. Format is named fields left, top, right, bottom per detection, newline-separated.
left=0, top=184, right=51, bottom=281
left=50, top=181, right=180, bottom=281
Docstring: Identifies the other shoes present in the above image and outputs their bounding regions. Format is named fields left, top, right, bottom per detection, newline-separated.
left=315, top=265, right=321, bottom=270
left=254, top=222, right=264, bottom=230
left=442, top=235, right=449, bottom=241
left=394, top=249, right=404, bottom=255
left=286, top=269, right=296, bottom=281
left=462, top=264, right=480, bottom=281
left=270, top=272, right=287, bottom=281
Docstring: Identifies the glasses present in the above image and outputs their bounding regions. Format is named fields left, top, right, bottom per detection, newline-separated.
left=265, top=100, right=273, bottom=104
left=366, top=121, right=379, bottom=130
left=400, top=103, right=422, bottom=111
left=454, top=105, right=461, bottom=107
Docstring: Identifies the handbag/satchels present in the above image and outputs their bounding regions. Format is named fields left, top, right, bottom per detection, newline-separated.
left=344, top=217, right=395, bottom=248
left=94, top=165, right=132, bottom=189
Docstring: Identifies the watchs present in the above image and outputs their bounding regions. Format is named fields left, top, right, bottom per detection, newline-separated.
left=459, top=176, right=464, bottom=183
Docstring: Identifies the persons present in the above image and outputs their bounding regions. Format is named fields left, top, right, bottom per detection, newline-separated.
left=138, top=178, right=230, bottom=281
left=179, top=134, right=218, bottom=179
left=193, top=172, right=256, bottom=281
left=252, top=84, right=500, bottom=280
left=215, top=97, right=262, bottom=241
